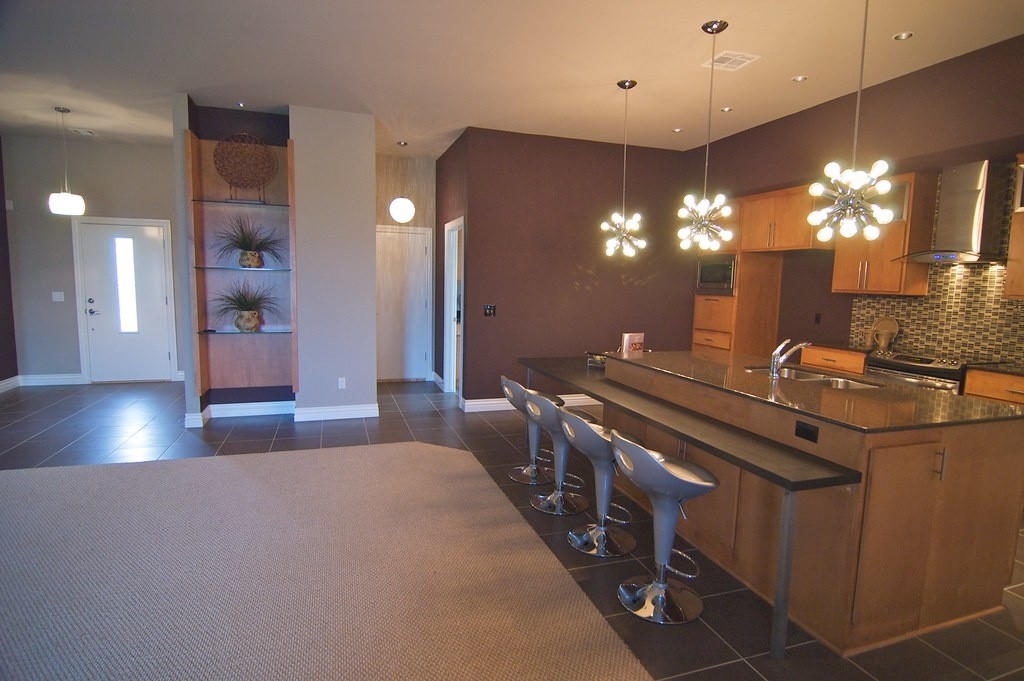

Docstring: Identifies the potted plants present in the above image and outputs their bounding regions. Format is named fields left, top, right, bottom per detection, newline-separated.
left=205, top=279, right=288, bottom=333
left=204, top=210, right=290, bottom=269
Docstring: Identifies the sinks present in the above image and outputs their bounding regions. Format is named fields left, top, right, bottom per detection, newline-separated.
left=745, top=367, right=835, bottom=380
left=799, top=380, right=882, bottom=389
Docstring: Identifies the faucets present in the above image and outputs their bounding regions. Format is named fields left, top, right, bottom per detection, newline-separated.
left=768, top=380, right=799, bottom=409
left=769, top=338, right=813, bottom=380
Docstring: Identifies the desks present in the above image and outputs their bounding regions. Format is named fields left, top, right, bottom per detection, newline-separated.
left=513, top=358, right=859, bottom=665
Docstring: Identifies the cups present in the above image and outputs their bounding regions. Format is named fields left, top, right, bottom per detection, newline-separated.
left=875, top=329, right=891, bottom=350
left=862, top=328, right=874, bottom=348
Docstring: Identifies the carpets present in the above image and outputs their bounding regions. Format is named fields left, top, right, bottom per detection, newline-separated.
left=1, top=442, right=655, bottom=681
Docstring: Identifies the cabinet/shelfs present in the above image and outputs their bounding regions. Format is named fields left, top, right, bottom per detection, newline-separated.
left=186, top=129, right=302, bottom=396
left=604, top=360, right=1023, bottom=660
left=687, top=170, right=935, bottom=361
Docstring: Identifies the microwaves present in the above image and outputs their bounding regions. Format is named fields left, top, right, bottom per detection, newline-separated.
left=696, top=254, right=736, bottom=296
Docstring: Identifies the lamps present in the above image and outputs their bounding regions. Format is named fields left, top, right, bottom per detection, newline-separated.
left=806, top=0, right=895, bottom=241
left=678, top=21, right=734, bottom=254
left=597, top=80, right=648, bottom=258
left=47, top=105, right=85, bottom=217
left=388, top=141, right=416, bottom=223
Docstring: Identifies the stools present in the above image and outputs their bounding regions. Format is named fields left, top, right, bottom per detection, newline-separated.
left=501, top=376, right=720, bottom=626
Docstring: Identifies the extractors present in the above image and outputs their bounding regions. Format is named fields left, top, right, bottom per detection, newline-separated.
left=889, top=160, right=1008, bottom=265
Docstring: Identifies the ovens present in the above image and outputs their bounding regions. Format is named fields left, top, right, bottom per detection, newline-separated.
left=865, top=365, right=963, bottom=396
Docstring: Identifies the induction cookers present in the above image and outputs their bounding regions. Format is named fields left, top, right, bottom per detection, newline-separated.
left=865, top=349, right=1016, bottom=381
